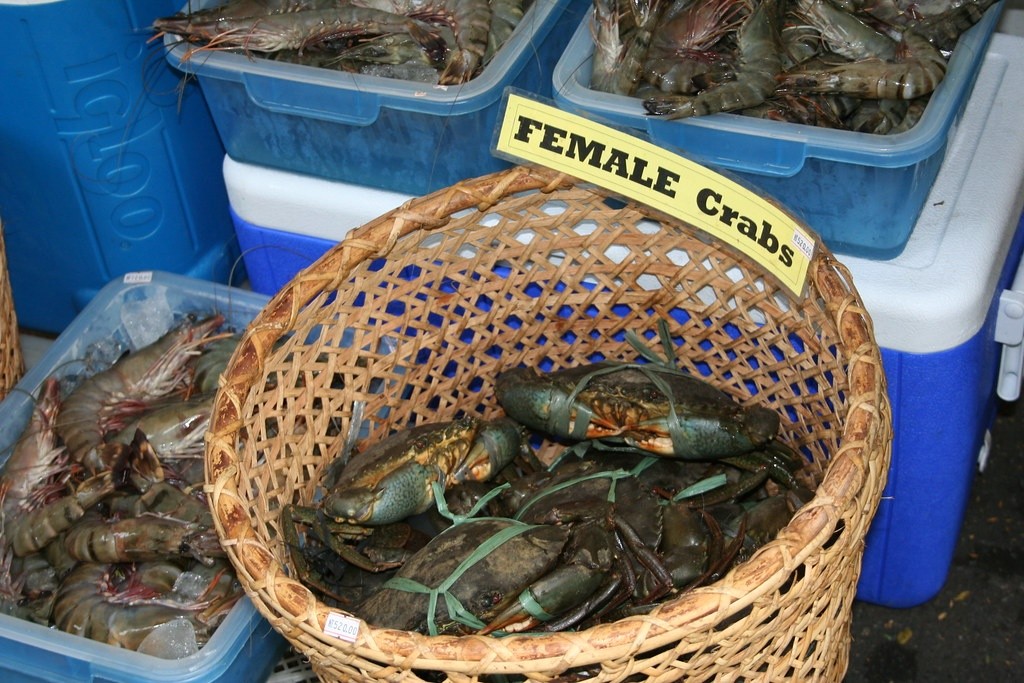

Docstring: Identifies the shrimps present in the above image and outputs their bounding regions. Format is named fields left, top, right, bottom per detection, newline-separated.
left=0, top=308, right=250, bottom=653
left=150, top=0, right=528, bottom=84
left=590, top=0, right=1001, bottom=145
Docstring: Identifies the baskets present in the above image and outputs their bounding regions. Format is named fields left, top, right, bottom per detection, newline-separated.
left=200, top=167, right=894, bottom=682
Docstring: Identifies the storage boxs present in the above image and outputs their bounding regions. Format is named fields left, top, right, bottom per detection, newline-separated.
left=2, top=2, right=251, bottom=340
left=554, top=1, right=1003, bottom=261
left=217, top=26, right=1024, bottom=606
left=2, top=267, right=417, bottom=681
left=165, top=0, right=592, bottom=197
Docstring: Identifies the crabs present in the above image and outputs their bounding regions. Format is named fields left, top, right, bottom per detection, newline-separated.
left=277, top=357, right=818, bottom=636
